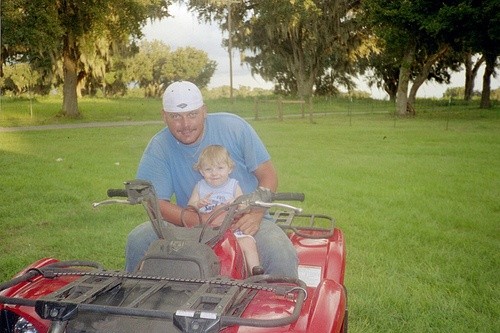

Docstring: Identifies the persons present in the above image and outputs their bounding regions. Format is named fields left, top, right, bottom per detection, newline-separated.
left=185, top=142, right=264, bottom=277
left=124, top=81, right=298, bottom=278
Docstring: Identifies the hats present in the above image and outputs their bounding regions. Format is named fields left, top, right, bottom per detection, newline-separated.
left=162, top=80, right=203, bottom=112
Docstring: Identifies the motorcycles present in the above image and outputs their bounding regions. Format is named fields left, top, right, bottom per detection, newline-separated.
left=0, top=177, right=350, bottom=333
